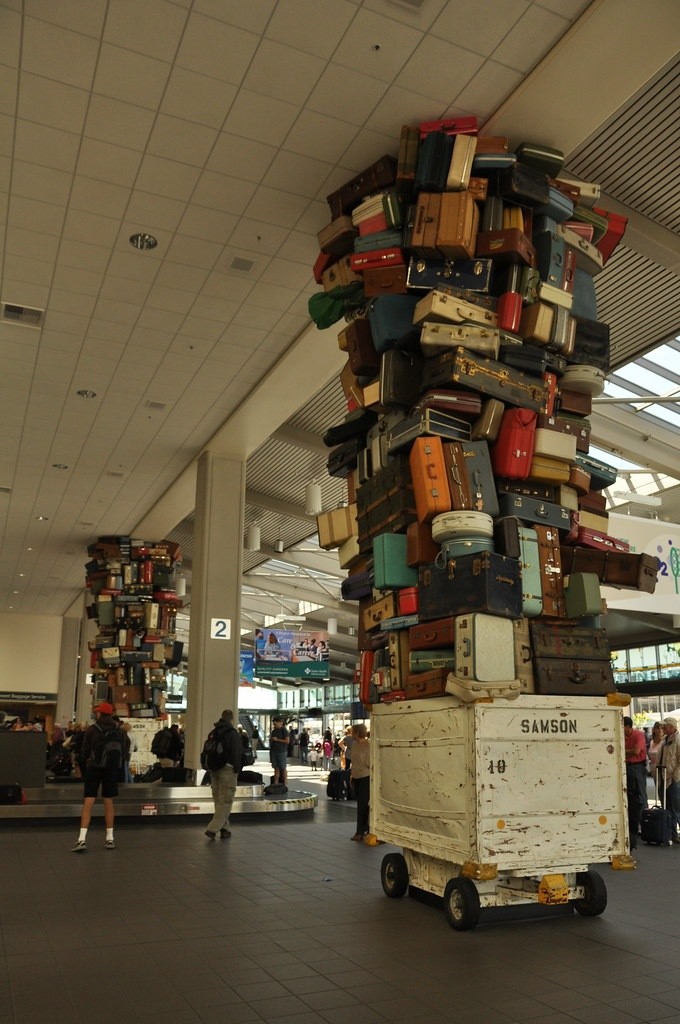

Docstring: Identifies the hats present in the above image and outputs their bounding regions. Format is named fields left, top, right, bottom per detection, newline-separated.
left=271, top=717, right=284, bottom=723
left=654, top=721, right=662, bottom=729
left=95, top=703, right=112, bottom=714
left=659, top=717, right=677, bottom=728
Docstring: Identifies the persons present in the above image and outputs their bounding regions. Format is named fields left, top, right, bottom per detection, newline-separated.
left=303, top=638, right=325, bottom=657
left=351, top=725, right=373, bottom=840
left=621, top=716, right=648, bottom=833
left=265, top=632, right=284, bottom=657
left=0, top=710, right=186, bottom=787
left=70, top=702, right=131, bottom=852
left=643, top=717, right=680, bottom=841
left=288, top=724, right=370, bottom=772
left=269, top=717, right=290, bottom=784
left=200, top=710, right=258, bottom=840
left=255, top=632, right=266, bottom=658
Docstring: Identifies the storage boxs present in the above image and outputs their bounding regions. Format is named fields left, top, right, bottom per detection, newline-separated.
left=568, top=546, right=659, bottom=595
left=556, top=316, right=610, bottom=373
left=358, top=454, right=418, bottom=556
left=328, top=155, right=399, bottom=221
left=417, top=346, right=549, bottom=415
left=490, top=163, right=549, bottom=213
left=529, top=625, right=615, bottom=695
left=89, top=712, right=163, bottom=767
left=405, top=256, right=492, bottom=293
left=387, top=407, right=472, bottom=457
left=533, top=524, right=568, bottom=618
left=368, top=695, right=636, bottom=881
left=417, top=549, right=523, bottom=620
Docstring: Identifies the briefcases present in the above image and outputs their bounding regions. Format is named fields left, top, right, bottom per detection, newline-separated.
left=85, top=534, right=184, bottom=718
left=307, top=114, right=661, bottom=706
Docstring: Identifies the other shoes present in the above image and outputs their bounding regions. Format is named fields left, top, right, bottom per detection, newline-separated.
left=220, top=828, right=232, bottom=838
left=205, top=830, right=216, bottom=838
left=350, top=833, right=363, bottom=840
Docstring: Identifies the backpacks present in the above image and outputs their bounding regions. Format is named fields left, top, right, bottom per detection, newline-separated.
left=201, top=727, right=237, bottom=770
left=91, top=724, right=122, bottom=771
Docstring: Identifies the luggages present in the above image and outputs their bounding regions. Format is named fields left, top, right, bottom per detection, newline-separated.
left=641, top=765, right=671, bottom=846
left=328, top=769, right=350, bottom=800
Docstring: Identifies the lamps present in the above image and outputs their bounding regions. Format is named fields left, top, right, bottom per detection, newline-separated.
left=613, top=452, right=662, bottom=506
left=175, top=572, right=186, bottom=597
left=338, top=487, right=348, bottom=509
left=347, top=618, right=355, bottom=636
left=176, top=663, right=184, bottom=675
left=327, top=612, right=338, bottom=636
left=274, top=528, right=283, bottom=553
left=271, top=677, right=278, bottom=688
left=306, top=478, right=322, bottom=516
left=295, top=676, right=302, bottom=685
left=247, top=520, right=260, bottom=552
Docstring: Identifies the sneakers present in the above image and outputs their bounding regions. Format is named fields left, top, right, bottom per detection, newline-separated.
left=71, top=840, right=87, bottom=851
left=105, top=840, right=114, bottom=848
